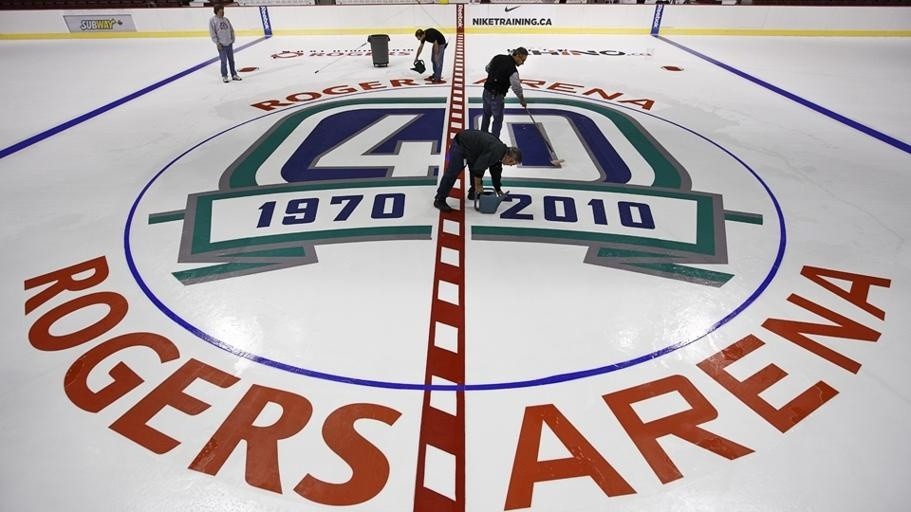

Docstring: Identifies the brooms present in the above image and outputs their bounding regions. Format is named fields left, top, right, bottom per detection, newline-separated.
left=525, top=108, right=564, bottom=166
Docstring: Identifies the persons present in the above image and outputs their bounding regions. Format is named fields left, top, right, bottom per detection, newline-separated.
left=432, top=128, right=522, bottom=213
left=479, top=46, right=529, bottom=139
left=207, top=2, right=243, bottom=83
left=412, top=26, right=449, bottom=84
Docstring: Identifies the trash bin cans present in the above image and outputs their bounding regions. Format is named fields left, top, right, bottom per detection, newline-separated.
left=367, top=35, right=390, bottom=67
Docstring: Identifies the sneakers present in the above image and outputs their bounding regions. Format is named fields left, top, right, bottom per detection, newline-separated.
left=434, top=197, right=451, bottom=212
left=232, top=75, right=241, bottom=80
left=468, top=189, right=480, bottom=200
left=223, top=76, right=228, bottom=83
left=424, top=76, right=441, bottom=84
